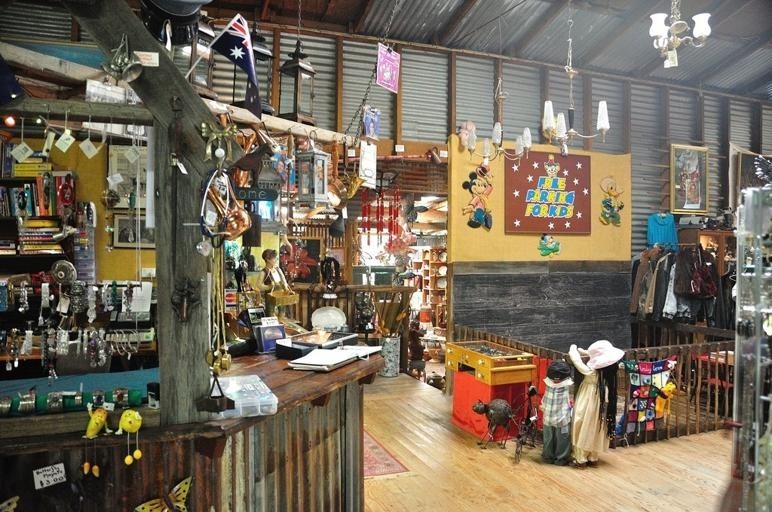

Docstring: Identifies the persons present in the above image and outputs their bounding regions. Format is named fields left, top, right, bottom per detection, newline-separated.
left=568, top=340, right=625, bottom=468
left=688, top=183, right=699, bottom=202
left=367, top=118, right=376, bottom=136
left=258, top=248, right=294, bottom=324
left=542, top=362, right=573, bottom=464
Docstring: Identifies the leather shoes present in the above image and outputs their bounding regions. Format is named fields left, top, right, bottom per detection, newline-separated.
left=568, top=460, right=599, bottom=470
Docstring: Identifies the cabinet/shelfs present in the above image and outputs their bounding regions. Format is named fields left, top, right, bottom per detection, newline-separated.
left=1, top=212, right=77, bottom=382
left=678, top=226, right=738, bottom=347
left=408, top=243, right=448, bottom=306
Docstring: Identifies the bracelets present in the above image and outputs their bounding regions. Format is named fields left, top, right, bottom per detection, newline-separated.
left=0, top=280, right=140, bottom=385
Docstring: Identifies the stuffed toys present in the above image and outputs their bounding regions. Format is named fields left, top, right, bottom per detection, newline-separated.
left=116, top=408, right=143, bottom=467
left=82, top=401, right=113, bottom=478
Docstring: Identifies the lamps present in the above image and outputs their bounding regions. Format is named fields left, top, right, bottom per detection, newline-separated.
left=274, top=0, right=317, bottom=126
left=456, top=0, right=537, bottom=166
left=229, top=10, right=276, bottom=116
left=529, top=1, right=612, bottom=154
left=295, top=136, right=329, bottom=209
left=168, top=11, right=220, bottom=100
left=647, top=1, right=712, bottom=69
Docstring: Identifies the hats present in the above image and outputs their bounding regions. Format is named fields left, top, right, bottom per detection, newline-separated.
left=587, top=340, right=625, bottom=370
left=547, top=360, right=571, bottom=380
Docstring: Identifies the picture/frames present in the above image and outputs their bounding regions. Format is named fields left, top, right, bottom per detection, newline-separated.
left=110, top=211, right=156, bottom=250
left=670, top=142, right=710, bottom=216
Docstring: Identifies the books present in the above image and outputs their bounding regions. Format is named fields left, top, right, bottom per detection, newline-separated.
left=287, top=345, right=383, bottom=374
left=104, top=327, right=159, bottom=349
left=0, top=143, right=75, bottom=256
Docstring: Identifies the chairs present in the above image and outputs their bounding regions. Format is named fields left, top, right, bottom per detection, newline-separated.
left=692, top=351, right=733, bottom=409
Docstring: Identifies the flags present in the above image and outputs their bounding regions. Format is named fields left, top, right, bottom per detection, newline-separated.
left=210, top=13, right=259, bottom=88
